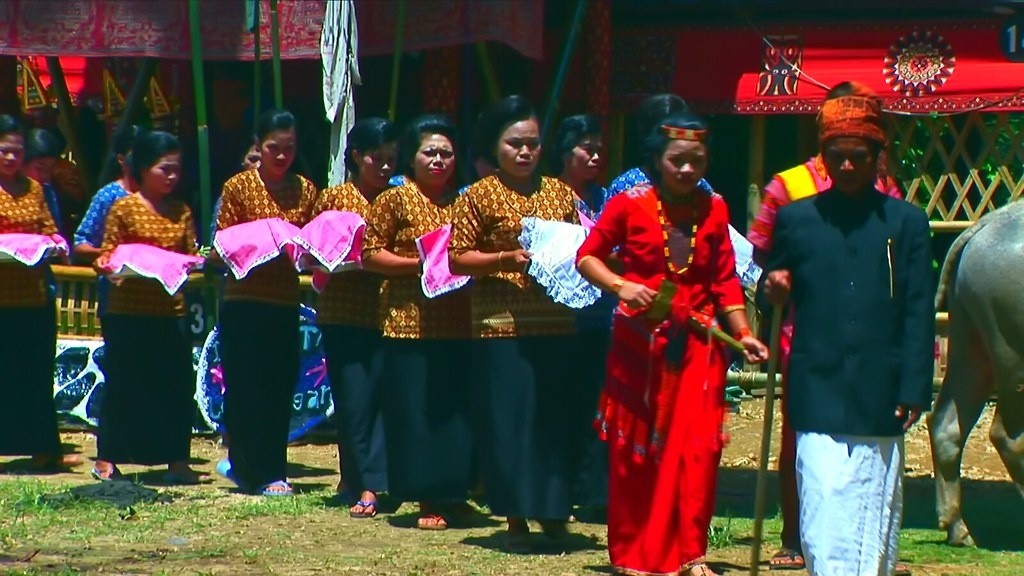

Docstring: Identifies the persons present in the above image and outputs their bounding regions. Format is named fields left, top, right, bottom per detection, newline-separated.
left=748, top=82, right=936, bottom=576
left=72, top=123, right=214, bottom=484
left=208, top=95, right=764, bottom=576
left=0, top=114, right=85, bottom=468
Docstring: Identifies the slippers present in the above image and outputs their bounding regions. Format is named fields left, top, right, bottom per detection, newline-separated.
left=416, top=512, right=449, bottom=530
left=894, top=569, right=911, bottom=576
left=770, top=550, right=805, bottom=570
left=164, top=473, right=214, bottom=484
left=92, top=468, right=134, bottom=484
left=689, top=563, right=716, bottom=576
left=349, top=500, right=376, bottom=518
left=255, top=481, right=294, bottom=496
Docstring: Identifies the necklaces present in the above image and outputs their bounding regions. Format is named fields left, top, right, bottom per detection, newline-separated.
left=656, top=188, right=699, bottom=276
left=494, top=174, right=538, bottom=217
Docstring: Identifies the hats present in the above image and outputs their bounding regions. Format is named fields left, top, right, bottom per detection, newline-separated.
left=819, top=92, right=887, bottom=145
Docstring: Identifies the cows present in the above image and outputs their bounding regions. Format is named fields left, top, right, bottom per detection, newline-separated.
left=925, top=198, right=1024, bottom=550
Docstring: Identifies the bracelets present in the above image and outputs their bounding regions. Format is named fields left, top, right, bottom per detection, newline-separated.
left=418, top=259, right=423, bottom=277
left=498, top=250, right=504, bottom=272
left=611, top=277, right=626, bottom=293
left=733, top=327, right=752, bottom=340
